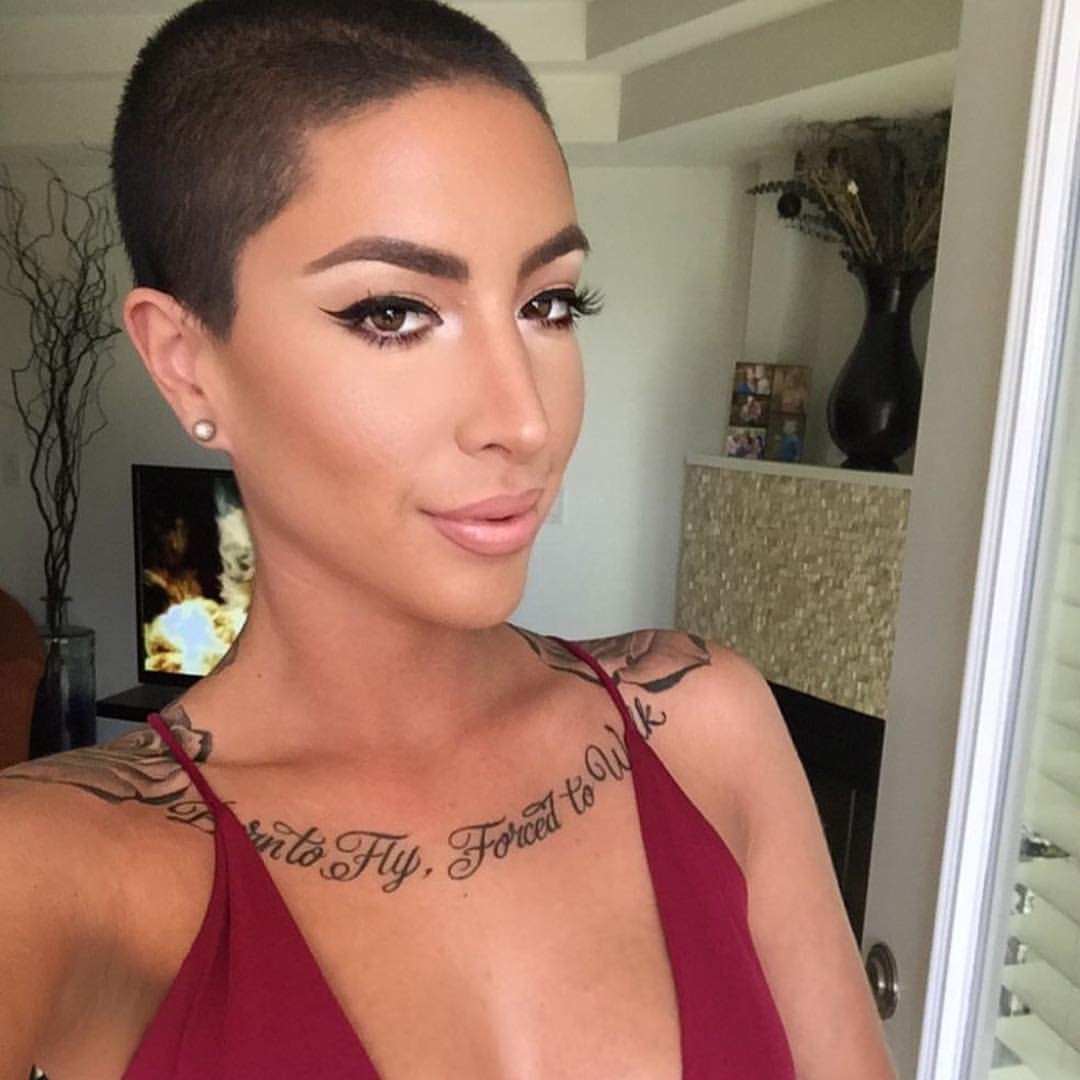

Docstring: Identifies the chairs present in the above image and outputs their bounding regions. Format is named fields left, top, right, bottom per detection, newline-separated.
left=0, top=590, right=45, bottom=769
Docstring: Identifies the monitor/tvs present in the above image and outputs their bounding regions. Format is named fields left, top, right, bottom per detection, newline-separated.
left=129, top=462, right=260, bottom=688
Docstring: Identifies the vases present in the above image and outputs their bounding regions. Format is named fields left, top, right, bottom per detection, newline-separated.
left=825, top=263, right=934, bottom=474
left=30, top=622, right=97, bottom=759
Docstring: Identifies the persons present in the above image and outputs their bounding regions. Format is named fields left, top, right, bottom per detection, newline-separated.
left=0, top=0, right=894, bottom=1080
left=739, top=365, right=770, bottom=395
left=740, top=396, right=755, bottom=426
left=727, top=431, right=764, bottom=459
left=782, top=372, right=802, bottom=412
left=774, top=418, right=800, bottom=460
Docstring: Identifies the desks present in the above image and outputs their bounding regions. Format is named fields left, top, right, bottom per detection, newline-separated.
left=96, top=686, right=191, bottom=723
